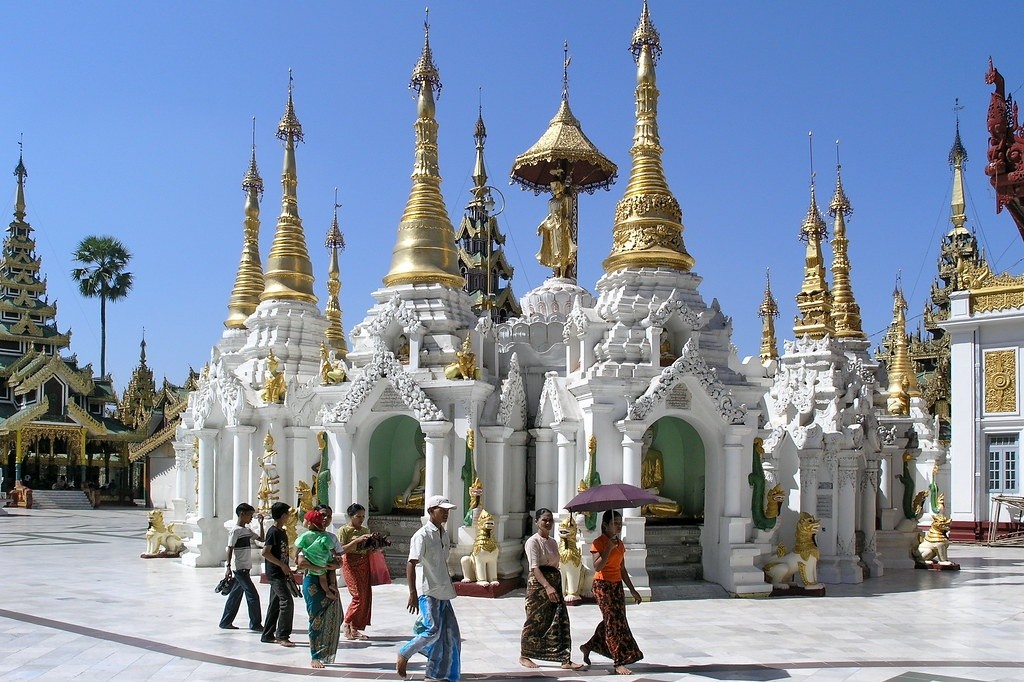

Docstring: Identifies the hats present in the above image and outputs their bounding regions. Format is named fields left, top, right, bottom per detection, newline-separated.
left=426, top=495, right=458, bottom=511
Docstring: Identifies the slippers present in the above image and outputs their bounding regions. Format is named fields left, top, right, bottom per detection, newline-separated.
left=284, top=576, right=303, bottom=598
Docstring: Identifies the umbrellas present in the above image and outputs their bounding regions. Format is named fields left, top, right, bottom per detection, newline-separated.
left=564, top=484, right=659, bottom=535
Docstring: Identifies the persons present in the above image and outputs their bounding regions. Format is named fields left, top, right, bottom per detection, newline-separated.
left=579, top=510, right=643, bottom=675
left=298, top=505, right=344, bottom=670
left=254, top=435, right=280, bottom=519
left=393, top=439, right=425, bottom=510
left=518, top=509, right=584, bottom=669
left=337, top=503, right=380, bottom=641
left=219, top=503, right=266, bottom=632
left=397, top=495, right=461, bottom=682
left=260, top=502, right=296, bottom=647
left=294, top=511, right=341, bottom=600
left=395, top=333, right=409, bottom=364
left=641, top=426, right=680, bottom=515
left=537, top=182, right=577, bottom=278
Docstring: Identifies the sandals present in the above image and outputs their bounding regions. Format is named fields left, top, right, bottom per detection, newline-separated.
left=214, top=577, right=237, bottom=596
